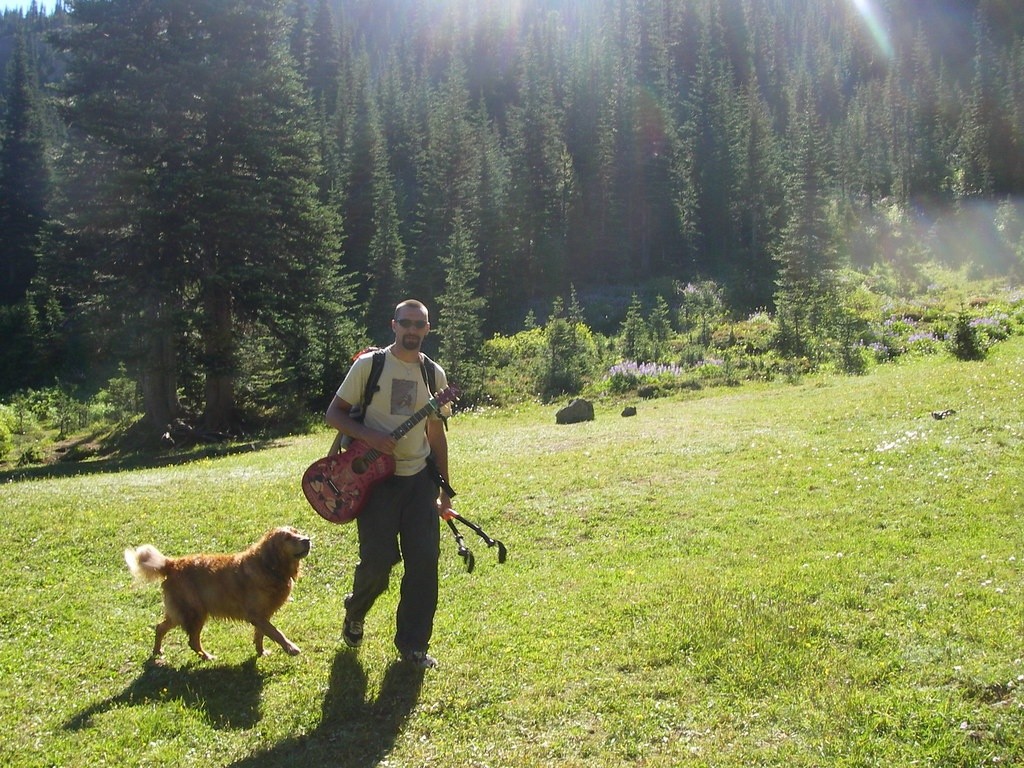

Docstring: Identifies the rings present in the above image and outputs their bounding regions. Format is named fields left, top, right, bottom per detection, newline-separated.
left=389, top=443, right=393, bottom=447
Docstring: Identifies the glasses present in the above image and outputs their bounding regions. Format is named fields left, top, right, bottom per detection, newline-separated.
left=396, top=318, right=428, bottom=329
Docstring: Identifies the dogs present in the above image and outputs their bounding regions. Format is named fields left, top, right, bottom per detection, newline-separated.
left=124, top=526, right=311, bottom=661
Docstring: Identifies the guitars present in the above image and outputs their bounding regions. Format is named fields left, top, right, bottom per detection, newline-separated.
left=300, top=380, right=463, bottom=526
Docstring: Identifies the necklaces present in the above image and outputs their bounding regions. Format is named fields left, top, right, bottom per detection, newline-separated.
left=393, top=353, right=418, bottom=375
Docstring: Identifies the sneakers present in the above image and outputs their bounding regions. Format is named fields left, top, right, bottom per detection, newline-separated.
left=342, top=594, right=363, bottom=647
left=400, top=651, right=438, bottom=668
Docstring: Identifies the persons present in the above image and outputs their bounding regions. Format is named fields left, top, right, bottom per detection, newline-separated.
left=325, top=299, right=452, bottom=673
